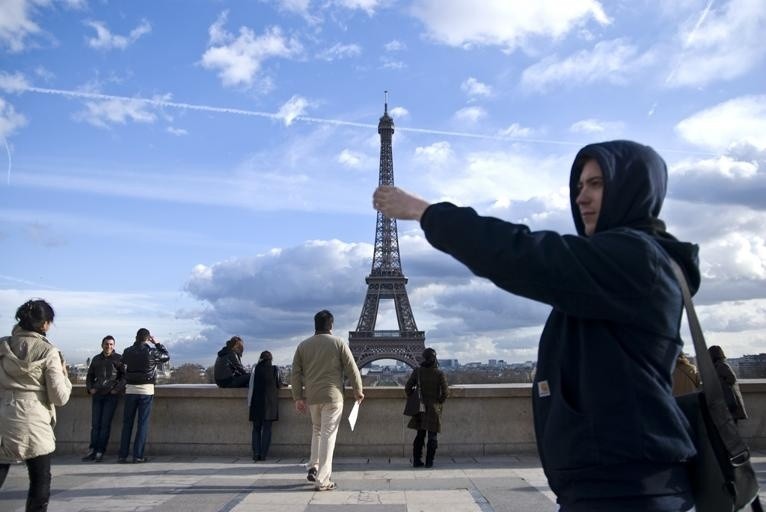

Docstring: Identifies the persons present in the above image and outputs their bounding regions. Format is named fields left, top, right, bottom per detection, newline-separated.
left=213, top=335, right=251, bottom=388
left=671, top=348, right=700, bottom=396
left=290, top=308, right=365, bottom=491
left=707, top=344, right=749, bottom=427
left=116, top=327, right=171, bottom=463
left=246, top=349, right=281, bottom=462
left=403, top=346, right=450, bottom=468
left=370, top=139, right=708, bottom=512
left=80, top=335, right=125, bottom=462
left=0, top=297, right=74, bottom=512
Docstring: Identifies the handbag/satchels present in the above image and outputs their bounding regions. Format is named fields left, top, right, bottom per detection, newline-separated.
left=404, top=367, right=426, bottom=416
left=668, top=256, right=766, bottom=512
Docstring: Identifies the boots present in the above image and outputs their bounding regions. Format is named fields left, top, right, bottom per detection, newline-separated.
left=425, top=439, right=437, bottom=468
left=413, top=440, right=424, bottom=467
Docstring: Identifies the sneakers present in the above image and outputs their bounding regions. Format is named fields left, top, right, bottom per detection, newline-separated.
left=133, top=456, right=145, bottom=462
left=81, top=451, right=96, bottom=462
left=307, top=467, right=318, bottom=481
left=315, top=481, right=336, bottom=491
left=117, top=457, right=127, bottom=464
left=94, top=452, right=104, bottom=462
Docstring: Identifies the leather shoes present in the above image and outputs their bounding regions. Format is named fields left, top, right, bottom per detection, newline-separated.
left=253, top=455, right=261, bottom=460
